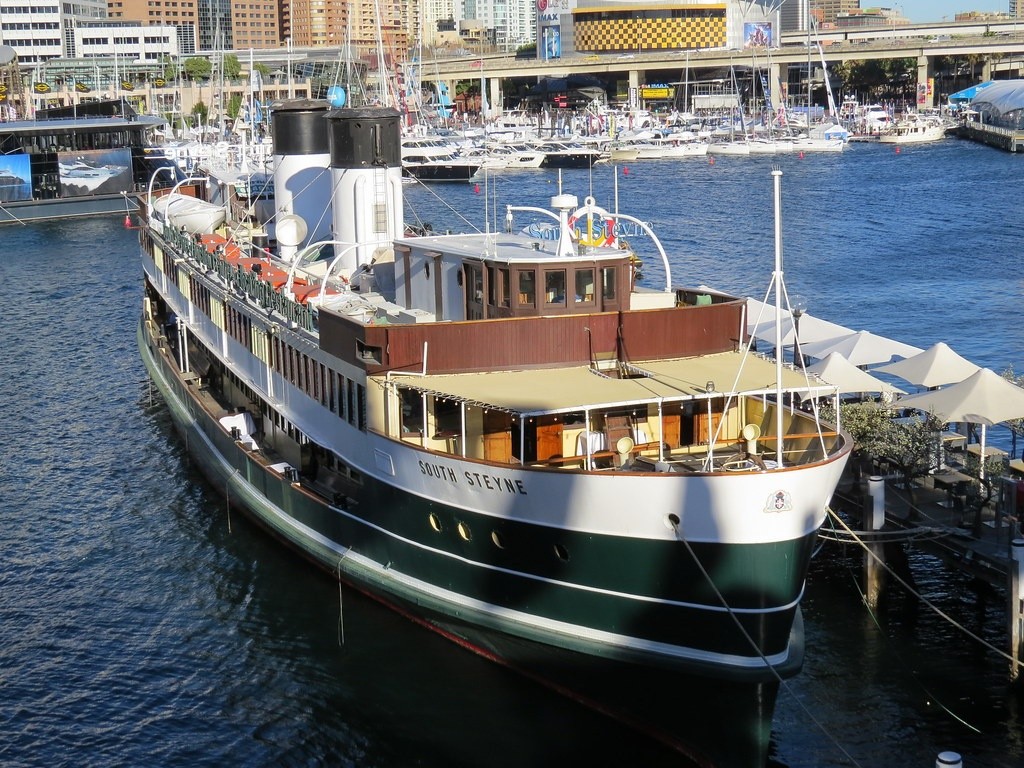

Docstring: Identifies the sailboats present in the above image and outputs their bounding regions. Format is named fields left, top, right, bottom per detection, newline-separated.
left=34, top=1, right=964, bottom=196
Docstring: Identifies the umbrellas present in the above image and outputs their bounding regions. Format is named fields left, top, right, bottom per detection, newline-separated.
left=691, top=283, right=1024, bottom=495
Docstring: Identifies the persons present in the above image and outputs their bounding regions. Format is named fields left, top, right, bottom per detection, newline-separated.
left=180, top=224, right=265, bottom=281
left=550, top=288, right=565, bottom=304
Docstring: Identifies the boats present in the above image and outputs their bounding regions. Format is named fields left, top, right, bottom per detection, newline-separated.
left=118, top=96, right=855, bottom=767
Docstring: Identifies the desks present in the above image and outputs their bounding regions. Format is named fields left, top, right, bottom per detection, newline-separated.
left=889, top=430, right=1024, bottom=528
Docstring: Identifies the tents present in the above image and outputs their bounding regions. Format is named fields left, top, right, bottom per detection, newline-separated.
left=946, top=77, right=1024, bottom=131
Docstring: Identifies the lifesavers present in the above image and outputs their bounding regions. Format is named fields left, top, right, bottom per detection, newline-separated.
left=567, top=205, right=615, bottom=248
